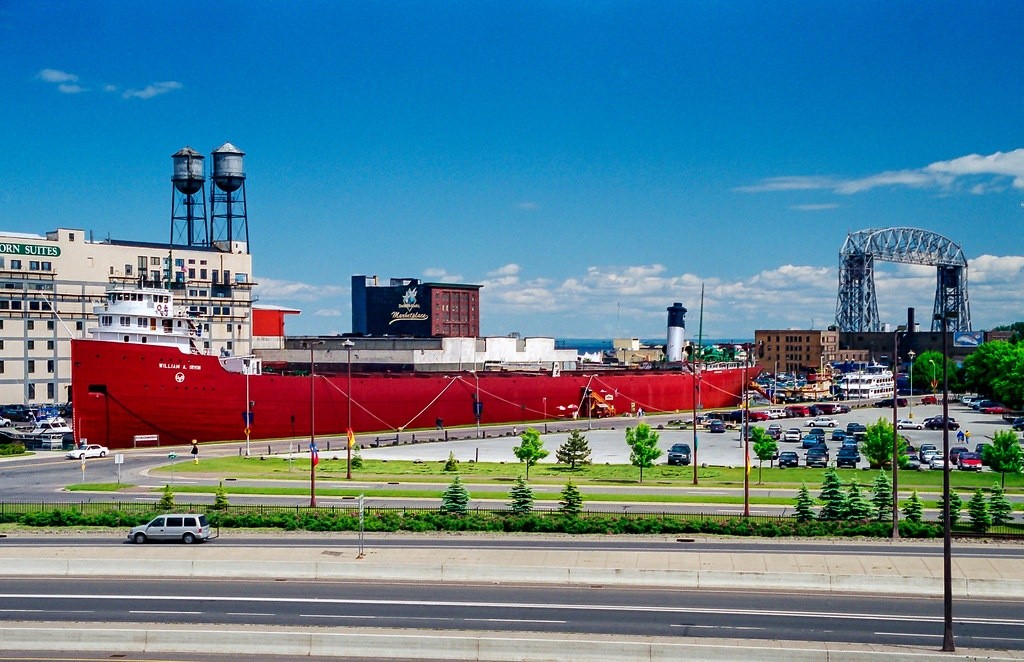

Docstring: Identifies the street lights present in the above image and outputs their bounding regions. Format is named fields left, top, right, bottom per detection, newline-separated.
left=893, top=313, right=955, bottom=652
left=311, top=339, right=354, bottom=508
left=908, top=350, right=915, bottom=420
left=928, top=360, right=936, bottom=397
left=689, top=343, right=756, bottom=517
left=244, top=359, right=250, bottom=456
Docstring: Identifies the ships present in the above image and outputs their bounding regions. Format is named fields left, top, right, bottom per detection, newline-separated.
left=71, top=247, right=765, bottom=450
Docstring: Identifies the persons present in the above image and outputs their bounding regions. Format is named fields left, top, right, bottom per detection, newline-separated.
left=957, top=431, right=964, bottom=441
left=965, top=430, right=970, bottom=444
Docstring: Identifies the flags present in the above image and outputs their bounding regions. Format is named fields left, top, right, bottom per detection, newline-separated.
left=181, top=265, right=187, bottom=272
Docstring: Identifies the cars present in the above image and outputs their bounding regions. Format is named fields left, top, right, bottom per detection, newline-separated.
left=738, top=396, right=1024, bottom=472
left=0, top=404, right=39, bottom=427
left=66, top=444, right=109, bottom=460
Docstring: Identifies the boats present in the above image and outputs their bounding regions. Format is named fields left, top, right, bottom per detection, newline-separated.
left=753, top=355, right=895, bottom=404
left=14, top=375, right=73, bottom=433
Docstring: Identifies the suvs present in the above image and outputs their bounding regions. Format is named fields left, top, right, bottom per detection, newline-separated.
left=667, top=444, right=691, bottom=465
left=804, top=448, right=829, bottom=467
left=696, top=416, right=726, bottom=433
left=127, top=514, right=211, bottom=544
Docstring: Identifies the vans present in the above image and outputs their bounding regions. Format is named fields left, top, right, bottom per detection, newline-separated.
left=787, top=405, right=809, bottom=417
left=957, top=452, right=982, bottom=471
left=705, top=411, right=736, bottom=429
left=816, top=404, right=841, bottom=414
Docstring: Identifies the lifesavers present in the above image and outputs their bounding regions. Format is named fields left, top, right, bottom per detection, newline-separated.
left=190, top=330, right=194, bottom=335
left=157, top=304, right=162, bottom=311
left=203, top=348, right=208, bottom=355
